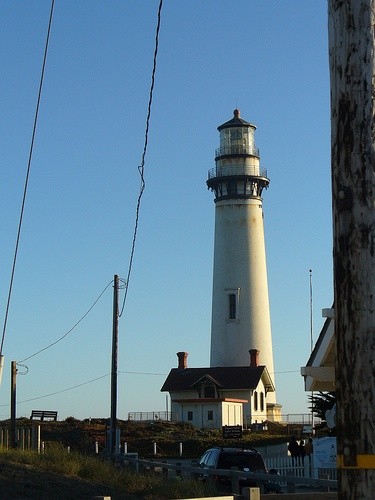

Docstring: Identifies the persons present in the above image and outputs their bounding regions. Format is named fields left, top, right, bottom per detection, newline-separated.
left=304, top=438, right=313, bottom=463
left=288, top=435, right=298, bottom=457
left=295, top=440, right=306, bottom=466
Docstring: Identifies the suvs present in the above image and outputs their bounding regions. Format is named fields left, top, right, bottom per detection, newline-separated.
left=189, top=446, right=267, bottom=495
